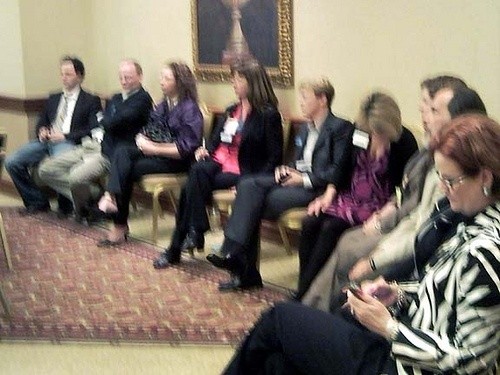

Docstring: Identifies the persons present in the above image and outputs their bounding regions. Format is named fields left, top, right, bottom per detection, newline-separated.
left=294, top=92, right=419, bottom=304
left=86, top=61, right=204, bottom=248
left=152, top=58, right=283, bottom=269
left=206, top=75, right=357, bottom=293
left=302, top=76, right=469, bottom=313
left=332, top=86, right=490, bottom=315
left=222, top=111, right=500, bottom=375
left=5, top=56, right=104, bottom=216
left=38, top=58, right=153, bottom=223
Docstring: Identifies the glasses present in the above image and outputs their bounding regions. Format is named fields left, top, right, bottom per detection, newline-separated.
left=436, top=170, right=478, bottom=191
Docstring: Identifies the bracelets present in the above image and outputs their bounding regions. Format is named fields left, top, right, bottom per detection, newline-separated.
left=368, top=256, right=376, bottom=271
left=386, top=321, right=400, bottom=343
left=388, top=280, right=403, bottom=314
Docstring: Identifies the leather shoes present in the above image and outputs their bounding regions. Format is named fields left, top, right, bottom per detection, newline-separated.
left=206, top=252, right=263, bottom=290
left=17, top=201, right=113, bottom=225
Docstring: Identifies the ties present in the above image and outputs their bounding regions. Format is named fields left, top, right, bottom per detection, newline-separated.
left=54, top=93, right=70, bottom=136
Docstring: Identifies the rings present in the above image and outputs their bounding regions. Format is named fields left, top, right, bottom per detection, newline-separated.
left=350, top=308, right=354, bottom=316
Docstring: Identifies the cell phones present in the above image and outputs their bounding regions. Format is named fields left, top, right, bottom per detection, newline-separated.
left=347, top=283, right=379, bottom=303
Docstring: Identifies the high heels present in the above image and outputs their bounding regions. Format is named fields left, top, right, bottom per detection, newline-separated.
left=97, top=226, right=129, bottom=248
left=153, top=228, right=204, bottom=269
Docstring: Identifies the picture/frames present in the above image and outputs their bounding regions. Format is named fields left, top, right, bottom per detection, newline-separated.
left=189, top=0, right=297, bottom=91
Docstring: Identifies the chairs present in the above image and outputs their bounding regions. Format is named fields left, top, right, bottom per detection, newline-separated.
left=135, top=115, right=332, bottom=257
